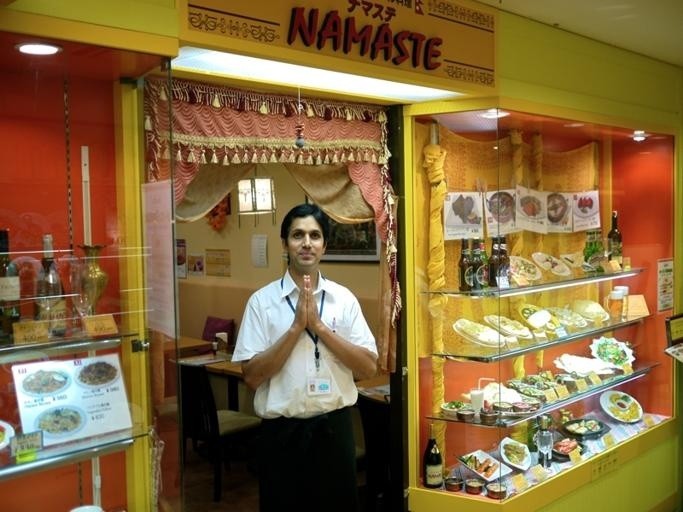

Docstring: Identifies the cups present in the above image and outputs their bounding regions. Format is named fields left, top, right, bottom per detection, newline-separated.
left=613, top=286, right=629, bottom=318
left=603, top=291, right=624, bottom=322
left=468, top=387, right=484, bottom=414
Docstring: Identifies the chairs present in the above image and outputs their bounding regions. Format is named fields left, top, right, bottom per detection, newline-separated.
left=182, top=376, right=262, bottom=504
left=200, top=316, right=241, bottom=410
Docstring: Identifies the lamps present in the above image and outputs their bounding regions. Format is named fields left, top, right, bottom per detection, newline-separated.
left=236, top=164, right=279, bottom=230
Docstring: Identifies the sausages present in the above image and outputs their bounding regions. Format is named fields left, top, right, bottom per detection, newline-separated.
left=485, top=464, right=497, bottom=478
left=476, top=457, right=490, bottom=474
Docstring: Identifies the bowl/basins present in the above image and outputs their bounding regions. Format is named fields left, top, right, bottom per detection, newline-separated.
left=513, top=401, right=528, bottom=411
left=463, top=479, right=484, bottom=494
left=456, top=409, right=476, bottom=420
left=487, top=483, right=507, bottom=499
left=445, top=478, right=464, bottom=492
left=479, top=411, right=500, bottom=423
left=492, top=402, right=513, bottom=413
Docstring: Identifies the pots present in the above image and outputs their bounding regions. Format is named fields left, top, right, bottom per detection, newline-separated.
left=487, top=192, right=515, bottom=223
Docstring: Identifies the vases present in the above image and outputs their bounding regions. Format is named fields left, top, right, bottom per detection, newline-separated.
left=77, top=242, right=110, bottom=317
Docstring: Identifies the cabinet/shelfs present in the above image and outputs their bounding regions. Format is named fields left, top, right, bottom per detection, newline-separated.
left=403, top=96, right=682, bottom=511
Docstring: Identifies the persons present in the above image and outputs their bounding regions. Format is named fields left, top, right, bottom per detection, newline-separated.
left=231, top=204, right=380, bottom=512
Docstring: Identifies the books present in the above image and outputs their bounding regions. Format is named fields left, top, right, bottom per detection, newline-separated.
left=13, top=353, right=132, bottom=447
left=179, top=357, right=225, bottom=366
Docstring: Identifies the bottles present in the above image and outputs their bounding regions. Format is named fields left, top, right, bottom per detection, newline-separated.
left=499, top=239, right=511, bottom=288
left=538, top=416, right=552, bottom=468
left=471, top=238, right=483, bottom=288
left=489, top=238, right=498, bottom=287
left=36, top=235, right=65, bottom=336
left=609, top=211, right=622, bottom=267
left=584, top=231, right=604, bottom=273
left=479, top=239, right=489, bottom=289
left=459, top=240, right=471, bottom=291
left=424, top=424, right=443, bottom=488
left=1, top=229, right=20, bottom=344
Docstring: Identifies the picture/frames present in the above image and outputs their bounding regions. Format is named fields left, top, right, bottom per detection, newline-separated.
left=304, top=193, right=382, bottom=264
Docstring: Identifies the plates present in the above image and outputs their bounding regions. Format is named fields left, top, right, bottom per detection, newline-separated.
left=499, top=436, right=533, bottom=472
left=0, top=419, right=16, bottom=450
left=75, top=367, right=121, bottom=388
left=548, top=192, right=568, bottom=223
left=509, top=255, right=541, bottom=281
left=441, top=403, right=470, bottom=413
left=518, top=194, right=546, bottom=220
left=34, top=405, right=87, bottom=438
left=599, top=390, right=642, bottom=423
left=453, top=323, right=505, bottom=348
left=21, top=370, right=72, bottom=397
left=455, top=449, right=514, bottom=482
left=557, top=419, right=611, bottom=439
left=564, top=302, right=609, bottom=323
left=532, top=252, right=570, bottom=277
left=517, top=303, right=560, bottom=335
left=589, top=335, right=636, bottom=369
left=445, top=196, right=481, bottom=227
left=484, top=315, right=534, bottom=340
left=573, top=203, right=598, bottom=218
left=552, top=356, right=614, bottom=377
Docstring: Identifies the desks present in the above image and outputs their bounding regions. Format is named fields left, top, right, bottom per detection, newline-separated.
left=178, top=330, right=210, bottom=360
left=351, top=375, right=393, bottom=411
left=187, top=346, right=270, bottom=412
